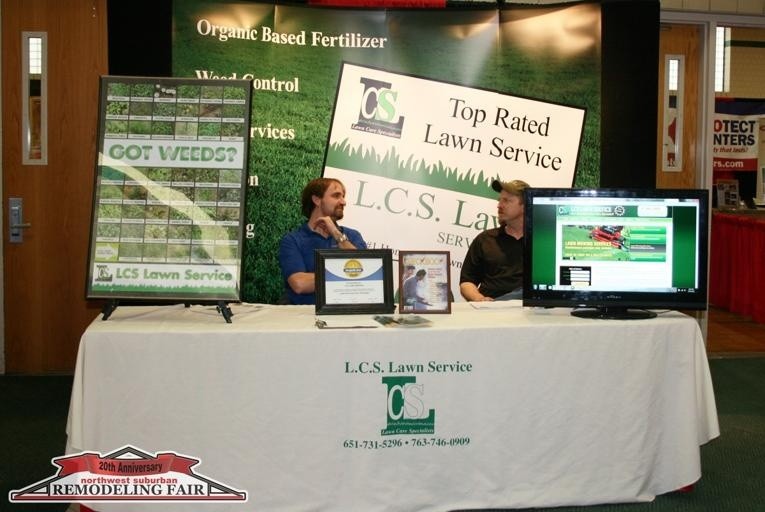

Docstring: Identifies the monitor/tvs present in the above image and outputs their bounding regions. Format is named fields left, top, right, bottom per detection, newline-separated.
left=524, top=187, right=709, bottom=320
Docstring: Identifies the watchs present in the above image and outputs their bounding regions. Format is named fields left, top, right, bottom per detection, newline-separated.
left=335, top=233, right=350, bottom=242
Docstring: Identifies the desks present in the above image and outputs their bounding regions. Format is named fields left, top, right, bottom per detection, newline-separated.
left=65, top=303, right=722, bottom=511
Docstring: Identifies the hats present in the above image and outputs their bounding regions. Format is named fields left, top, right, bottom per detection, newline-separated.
left=492, top=180, right=529, bottom=195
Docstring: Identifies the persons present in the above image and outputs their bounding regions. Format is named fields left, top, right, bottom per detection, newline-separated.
left=460, top=180, right=531, bottom=302
left=278, top=179, right=368, bottom=304
left=404, top=270, right=434, bottom=308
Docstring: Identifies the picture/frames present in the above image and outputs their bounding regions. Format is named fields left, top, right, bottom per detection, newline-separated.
left=314, top=247, right=395, bottom=315
left=397, top=250, right=452, bottom=315
